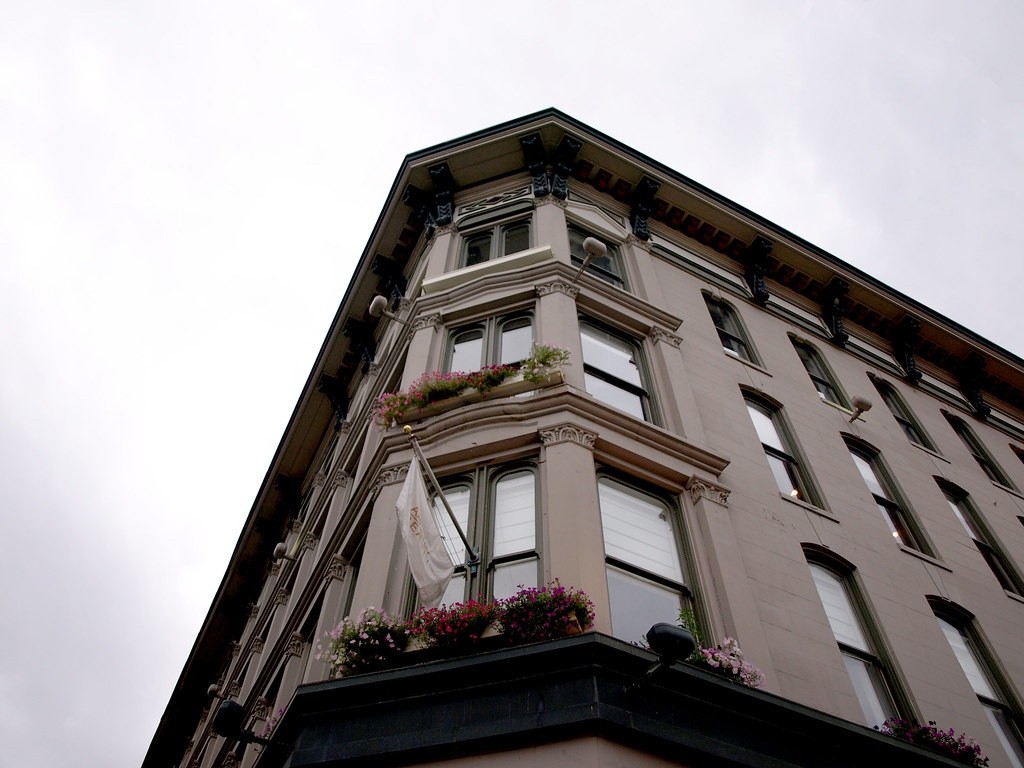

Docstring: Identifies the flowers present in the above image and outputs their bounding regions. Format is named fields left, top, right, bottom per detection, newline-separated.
left=370, top=343, right=571, bottom=433
left=258, top=705, right=285, bottom=739
left=630, top=605, right=766, bottom=688
left=315, top=576, right=596, bottom=680
left=874, top=718, right=989, bottom=767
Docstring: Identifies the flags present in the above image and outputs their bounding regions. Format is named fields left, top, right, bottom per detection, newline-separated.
left=393, top=451, right=455, bottom=617
